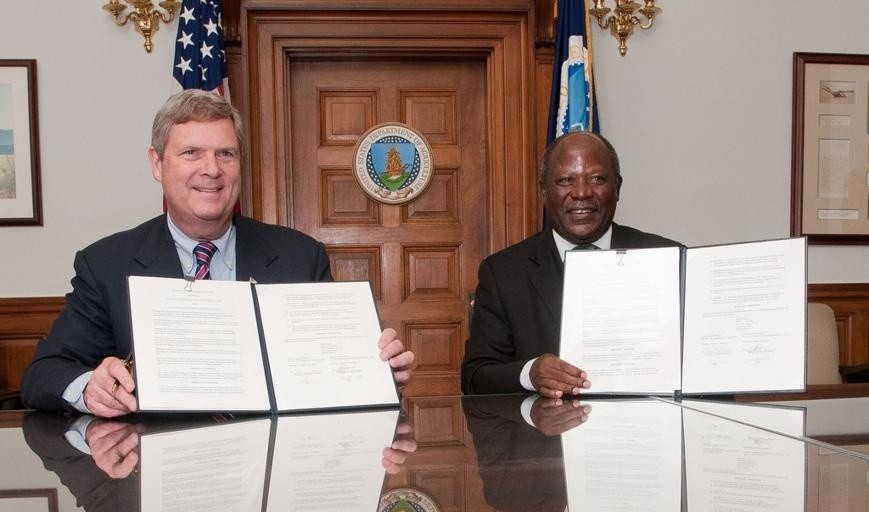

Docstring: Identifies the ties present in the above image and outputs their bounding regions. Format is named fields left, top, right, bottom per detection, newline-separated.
left=191, top=242, right=217, bottom=280
left=574, top=243, right=598, bottom=250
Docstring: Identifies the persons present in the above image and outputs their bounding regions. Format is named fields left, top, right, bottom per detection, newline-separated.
left=459, top=131, right=691, bottom=402
left=19, top=404, right=419, bottom=512
left=460, top=393, right=593, bottom=512
left=15, top=88, right=417, bottom=424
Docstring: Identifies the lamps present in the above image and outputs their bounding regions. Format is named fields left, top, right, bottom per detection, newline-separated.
left=590, top=0, right=662, bottom=56
left=102, top=0, right=181, bottom=53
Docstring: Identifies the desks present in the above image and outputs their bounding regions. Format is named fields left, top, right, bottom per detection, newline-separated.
left=0, top=395, right=868, bottom=512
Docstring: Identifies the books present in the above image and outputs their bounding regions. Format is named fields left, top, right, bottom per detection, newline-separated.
left=122, top=269, right=404, bottom=416
left=557, top=233, right=809, bottom=404
left=137, top=406, right=401, bottom=511
left=554, top=390, right=812, bottom=512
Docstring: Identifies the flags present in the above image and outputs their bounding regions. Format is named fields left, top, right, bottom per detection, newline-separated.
left=163, top=0, right=246, bottom=222
left=541, top=1, right=603, bottom=233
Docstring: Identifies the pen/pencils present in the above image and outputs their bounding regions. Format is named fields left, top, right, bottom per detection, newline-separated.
left=112, top=349, right=134, bottom=393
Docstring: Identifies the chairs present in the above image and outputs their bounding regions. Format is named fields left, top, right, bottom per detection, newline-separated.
left=807, top=303, right=845, bottom=385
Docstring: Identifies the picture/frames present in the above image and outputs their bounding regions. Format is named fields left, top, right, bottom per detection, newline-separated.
left=0, top=60, right=43, bottom=227
left=0, top=488, right=58, bottom=512
left=790, top=52, right=869, bottom=245
